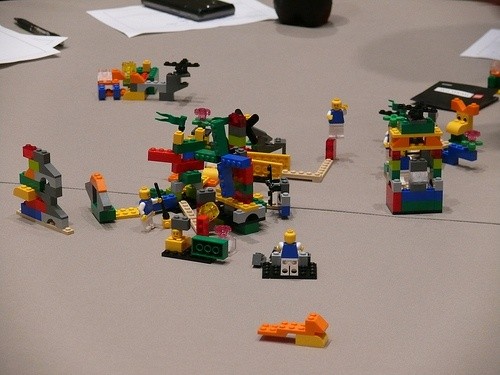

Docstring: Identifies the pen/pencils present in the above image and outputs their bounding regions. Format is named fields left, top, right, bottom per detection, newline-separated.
left=13, top=17, right=64, bottom=45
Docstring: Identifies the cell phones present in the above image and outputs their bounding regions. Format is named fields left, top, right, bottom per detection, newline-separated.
left=141, top=0, right=236, bottom=21
